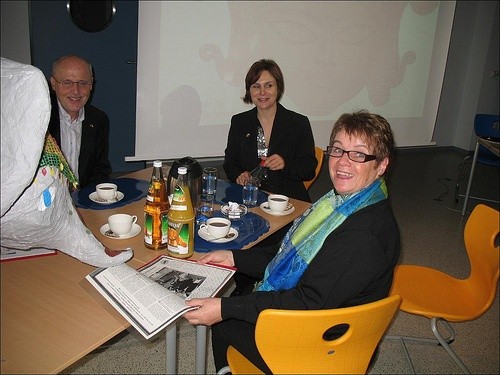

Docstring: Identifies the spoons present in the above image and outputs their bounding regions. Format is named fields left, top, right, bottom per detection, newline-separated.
left=95, top=198, right=118, bottom=202
left=104, top=229, right=120, bottom=237
left=208, top=233, right=235, bottom=242
left=264, top=203, right=293, bottom=210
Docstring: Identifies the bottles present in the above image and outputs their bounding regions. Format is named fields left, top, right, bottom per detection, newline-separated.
left=167, top=167, right=196, bottom=259
left=143, top=160, right=170, bottom=250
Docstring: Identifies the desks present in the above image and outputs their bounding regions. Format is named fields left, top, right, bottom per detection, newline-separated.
left=62, top=165, right=314, bottom=375
left=0, top=233, right=179, bottom=375
left=461, top=136, right=500, bottom=218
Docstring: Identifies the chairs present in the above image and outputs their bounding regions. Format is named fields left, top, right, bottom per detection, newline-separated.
left=215, top=294, right=401, bottom=375
left=456, top=113, right=500, bottom=203
left=378, top=202, right=500, bottom=375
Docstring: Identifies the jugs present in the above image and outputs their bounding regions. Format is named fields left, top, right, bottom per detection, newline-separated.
left=167, top=156, right=203, bottom=217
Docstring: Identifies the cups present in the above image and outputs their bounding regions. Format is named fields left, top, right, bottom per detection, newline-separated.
left=267, top=194, right=289, bottom=211
left=108, top=213, right=138, bottom=233
left=200, top=217, right=231, bottom=237
left=202, top=167, right=218, bottom=198
left=243, top=178, right=258, bottom=204
left=195, top=194, right=215, bottom=226
left=96, top=183, right=118, bottom=199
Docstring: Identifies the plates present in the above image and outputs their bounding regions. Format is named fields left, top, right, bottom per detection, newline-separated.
left=198, top=226, right=239, bottom=243
left=260, top=202, right=296, bottom=215
left=99, top=223, right=141, bottom=240
left=89, top=191, right=125, bottom=203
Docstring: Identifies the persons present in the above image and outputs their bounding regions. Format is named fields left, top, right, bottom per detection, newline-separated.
left=44, top=56, right=110, bottom=193
left=181, top=111, right=402, bottom=375
left=223, top=59, right=318, bottom=287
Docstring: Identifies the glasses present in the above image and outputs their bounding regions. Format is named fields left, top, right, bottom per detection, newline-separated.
left=327, top=146, right=377, bottom=163
left=51, top=75, right=92, bottom=90
